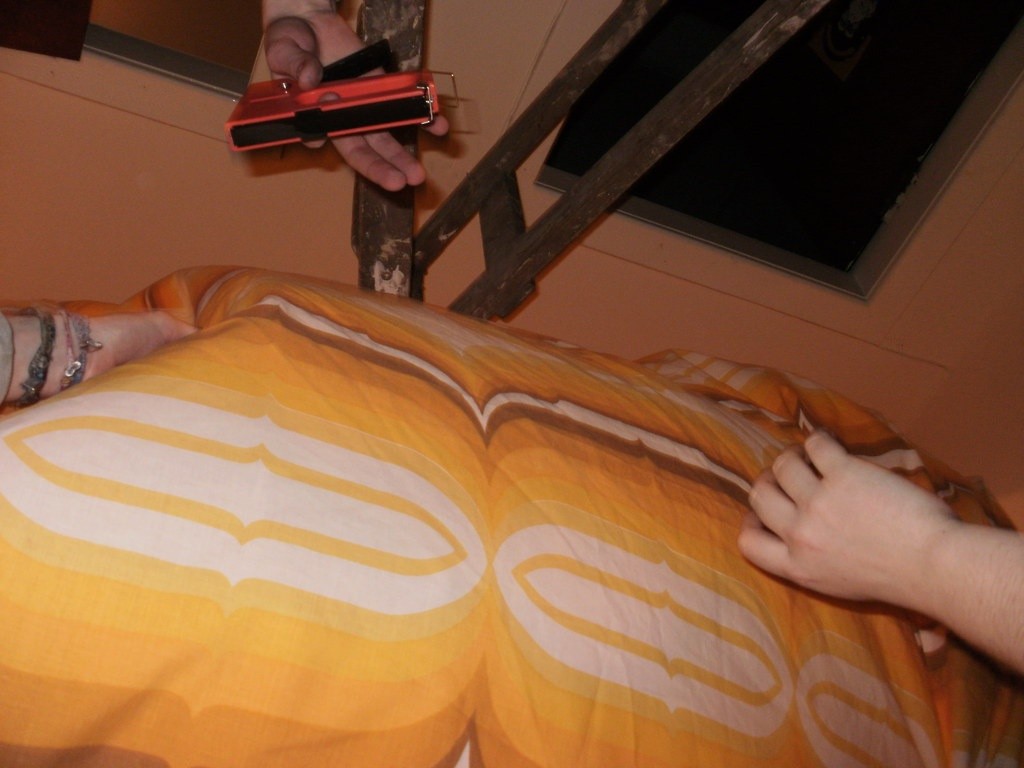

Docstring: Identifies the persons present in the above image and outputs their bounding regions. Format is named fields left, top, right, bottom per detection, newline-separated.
left=261, top=0, right=449, bottom=191
left=0, top=305, right=203, bottom=406
left=738, top=428, right=1024, bottom=676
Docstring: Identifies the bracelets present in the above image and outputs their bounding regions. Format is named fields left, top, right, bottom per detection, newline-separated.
left=56, top=310, right=102, bottom=391
left=17, top=306, right=56, bottom=407
